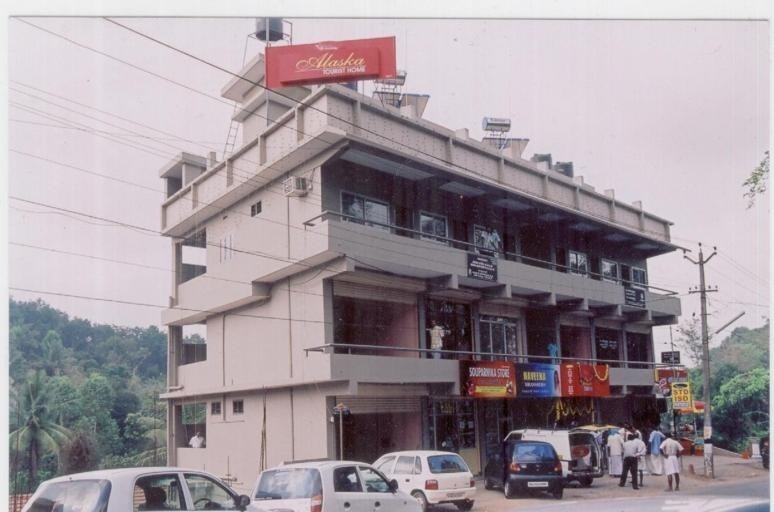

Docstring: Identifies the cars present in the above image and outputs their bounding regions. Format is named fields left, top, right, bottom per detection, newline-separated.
left=19, top=466, right=267, bottom=512
left=249, top=458, right=424, bottom=512
left=484, top=439, right=562, bottom=499
left=570, top=423, right=633, bottom=470
left=372, top=450, right=477, bottom=511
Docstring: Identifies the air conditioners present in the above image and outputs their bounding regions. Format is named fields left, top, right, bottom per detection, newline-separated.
left=281, top=176, right=308, bottom=199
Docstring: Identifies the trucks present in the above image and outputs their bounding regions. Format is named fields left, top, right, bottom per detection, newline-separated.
left=504, top=422, right=604, bottom=487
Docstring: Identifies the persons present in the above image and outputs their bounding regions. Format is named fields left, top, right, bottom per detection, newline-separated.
left=188, top=431, right=206, bottom=448
left=546, top=337, right=560, bottom=364
left=429, top=319, right=445, bottom=359
left=596, top=419, right=693, bottom=493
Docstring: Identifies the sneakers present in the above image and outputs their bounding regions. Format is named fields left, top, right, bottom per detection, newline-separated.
left=663, top=486, right=680, bottom=492
left=616, top=481, right=644, bottom=490
